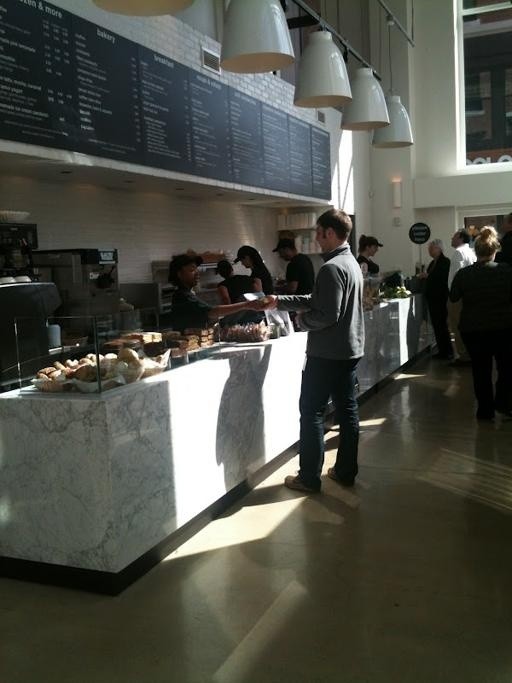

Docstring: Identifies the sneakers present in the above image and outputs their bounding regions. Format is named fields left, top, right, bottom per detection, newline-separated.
left=432, top=353, right=454, bottom=360
left=328, top=468, right=354, bottom=485
left=477, top=403, right=495, bottom=420
left=285, top=475, right=319, bottom=493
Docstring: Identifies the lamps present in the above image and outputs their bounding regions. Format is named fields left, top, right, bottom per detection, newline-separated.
left=340, top=0, right=390, bottom=133
left=94, top=0, right=196, bottom=18
left=219, top=0, right=296, bottom=75
left=293, top=1, right=354, bottom=108
left=371, top=16, right=412, bottom=153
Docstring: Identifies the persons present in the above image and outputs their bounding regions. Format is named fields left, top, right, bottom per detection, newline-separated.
left=273, top=238, right=315, bottom=295
left=267, top=209, right=364, bottom=491
left=214, top=262, right=248, bottom=305
left=234, top=246, right=273, bottom=296
left=417, top=215, right=511, bottom=421
left=357, top=235, right=383, bottom=278
left=171, top=255, right=266, bottom=336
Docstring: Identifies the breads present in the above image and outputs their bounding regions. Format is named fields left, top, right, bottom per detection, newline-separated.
left=102, top=327, right=214, bottom=356
left=36, top=347, right=144, bottom=381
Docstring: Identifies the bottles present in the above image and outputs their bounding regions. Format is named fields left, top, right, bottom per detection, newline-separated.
left=2, top=236, right=33, bottom=267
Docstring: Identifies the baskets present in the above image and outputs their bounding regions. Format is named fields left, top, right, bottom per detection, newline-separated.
left=30, top=355, right=168, bottom=393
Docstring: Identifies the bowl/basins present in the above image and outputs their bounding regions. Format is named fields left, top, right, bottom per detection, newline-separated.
left=0, top=276, right=32, bottom=284
left=0, top=210, right=30, bottom=223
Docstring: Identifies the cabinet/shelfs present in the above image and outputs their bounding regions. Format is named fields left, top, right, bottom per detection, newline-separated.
left=277, top=215, right=321, bottom=255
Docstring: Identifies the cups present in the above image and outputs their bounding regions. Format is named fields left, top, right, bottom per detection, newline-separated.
left=47, top=324, right=61, bottom=347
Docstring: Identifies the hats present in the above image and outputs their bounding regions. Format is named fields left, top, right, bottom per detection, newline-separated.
left=365, top=236, right=383, bottom=247
left=170, top=254, right=203, bottom=272
left=234, top=246, right=253, bottom=263
left=272, top=239, right=295, bottom=252
